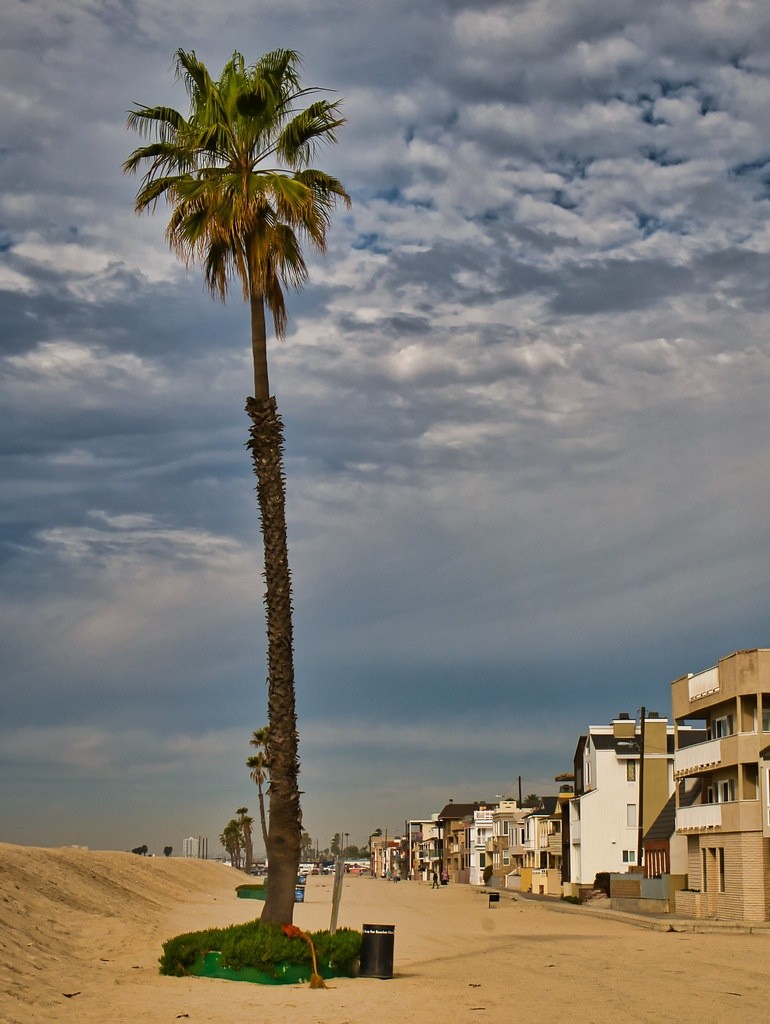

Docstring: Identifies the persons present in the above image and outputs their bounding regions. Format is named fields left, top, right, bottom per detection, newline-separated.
left=431, top=871, right=440, bottom=889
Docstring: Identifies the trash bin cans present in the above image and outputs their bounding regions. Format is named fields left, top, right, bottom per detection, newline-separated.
left=362, top=921, right=396, bottom=978
left=489, top=892, right=499, bottom=908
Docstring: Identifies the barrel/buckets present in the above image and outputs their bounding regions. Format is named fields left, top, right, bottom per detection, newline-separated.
left=358, top=923, right=396, bottom=979
left=489, top=894, right=499, bottom=908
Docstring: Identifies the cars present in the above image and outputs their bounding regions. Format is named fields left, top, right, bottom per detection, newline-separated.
left=299, top=870, right=309, bottom=877
left=321, top=870, right=328, bottom=876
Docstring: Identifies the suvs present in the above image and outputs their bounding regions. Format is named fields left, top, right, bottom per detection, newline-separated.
left=311, top=868, right=319, bottom=876
